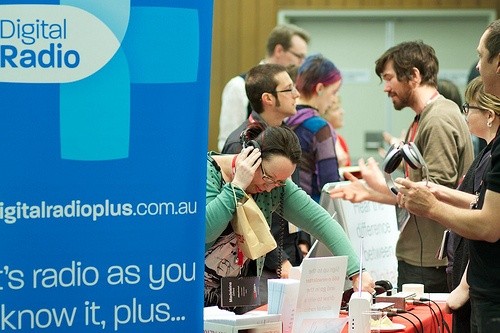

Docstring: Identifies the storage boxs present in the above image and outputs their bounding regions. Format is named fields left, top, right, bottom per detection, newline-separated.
left=204, top=314, right=282, bottom=333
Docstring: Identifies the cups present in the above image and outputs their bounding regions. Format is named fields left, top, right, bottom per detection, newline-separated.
left=363, top=311, right=380, bottom=333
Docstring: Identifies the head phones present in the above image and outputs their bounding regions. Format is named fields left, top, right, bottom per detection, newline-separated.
left=384, top=142, right=428, bottom=197
left=240, top=128, right=262, bottom=165
left=341, top=280, right=393, bottom=311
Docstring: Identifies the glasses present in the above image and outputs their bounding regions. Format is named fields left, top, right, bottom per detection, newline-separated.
left=461, top=101, right=491, bottom=115
left=259, top=163, right=286, bottom=189
left=269, top=83, right=296, bottom=94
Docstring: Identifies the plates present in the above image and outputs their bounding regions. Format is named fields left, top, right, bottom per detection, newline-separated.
left=371, top=323, right=406, bottom=331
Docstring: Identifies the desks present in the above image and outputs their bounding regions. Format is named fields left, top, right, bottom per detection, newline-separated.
left=244, top=292, right=455, bottom=333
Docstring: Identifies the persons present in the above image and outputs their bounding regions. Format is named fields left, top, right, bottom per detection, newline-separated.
left=206, top=126, right=376, bottom=298
left=222, top=64, right=309, bottom=281
left=435, top=79, right=462, bottom=110
left=398, top=19, right=500, bottom=333
left=283, top=55, right=341, bottom=258
left=414, top=78, right=500, bottom=333
left=323, top=95, right=350, bottom=169
left=219, top=24, right=310, bottom=151
left=328, top=41, right=475, bottom=296
